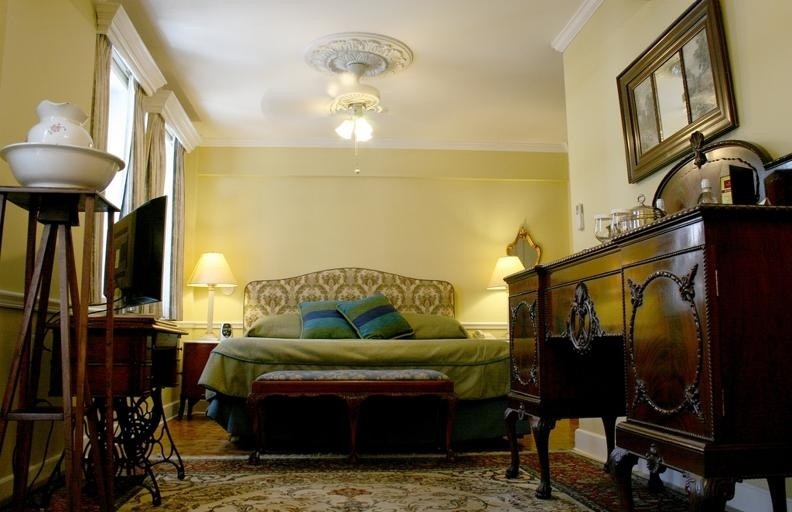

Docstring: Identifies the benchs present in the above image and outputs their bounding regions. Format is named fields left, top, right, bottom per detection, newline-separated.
left=244, top=370, right=458, bottom=465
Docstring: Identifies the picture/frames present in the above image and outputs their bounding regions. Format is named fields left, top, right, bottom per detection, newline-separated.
left=613, top=0, right=741, bottom=187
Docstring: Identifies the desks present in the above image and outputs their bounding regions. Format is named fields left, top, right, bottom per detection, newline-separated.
left=0, top=184, right=123, bottom=510
left=48, top=311, right=193, bottom=508
left=500, top=132, right=791, bottom=511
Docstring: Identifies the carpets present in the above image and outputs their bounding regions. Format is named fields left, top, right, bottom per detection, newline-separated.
left=13, top=452, right=746, bottom=511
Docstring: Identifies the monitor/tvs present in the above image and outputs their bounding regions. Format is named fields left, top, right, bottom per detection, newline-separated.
left=103, top=195, right=168, bottom=308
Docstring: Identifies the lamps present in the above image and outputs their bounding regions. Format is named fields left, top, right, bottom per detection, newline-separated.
left=485, top=254, right=528, bottom=294
left=301, top=29, right=416, bottom=179
left=185, top=249, right=240, bottom=345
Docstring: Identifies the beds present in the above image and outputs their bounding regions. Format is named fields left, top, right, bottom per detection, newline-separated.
left=196, top=266, right=533, bottom=451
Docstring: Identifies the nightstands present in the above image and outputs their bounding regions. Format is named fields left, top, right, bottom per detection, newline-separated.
left=176, top=341, right=222, bottom=424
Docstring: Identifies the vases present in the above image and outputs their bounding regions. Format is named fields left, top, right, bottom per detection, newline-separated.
left=22, top=96, right=98, bottom=153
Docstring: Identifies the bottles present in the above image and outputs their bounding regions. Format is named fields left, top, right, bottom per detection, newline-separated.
left=593, top=195, right=664, bottom=245
left=27, top=100, right=95, bottom=149
left=698, top=178, right=717, bottom=205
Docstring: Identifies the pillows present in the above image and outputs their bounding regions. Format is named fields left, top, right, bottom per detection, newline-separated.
left=245, top=291, right=470, bottom=341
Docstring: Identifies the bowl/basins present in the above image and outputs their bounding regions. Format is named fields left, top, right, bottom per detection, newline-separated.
left=1, top=143, right=126, bottom=194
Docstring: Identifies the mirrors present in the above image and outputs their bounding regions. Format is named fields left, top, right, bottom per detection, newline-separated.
left=503, top=224, right=544, bottom=271
